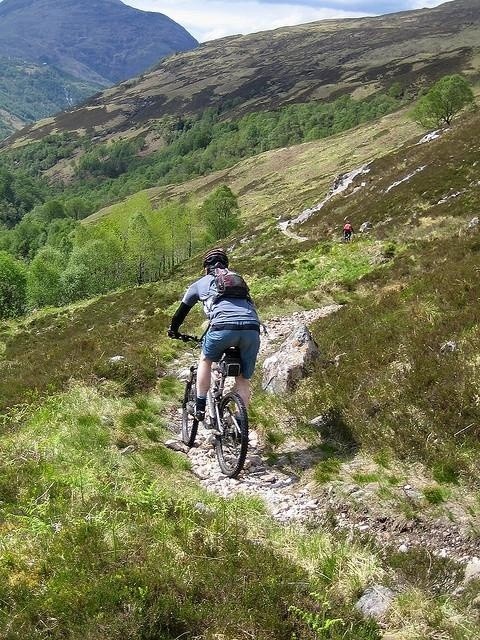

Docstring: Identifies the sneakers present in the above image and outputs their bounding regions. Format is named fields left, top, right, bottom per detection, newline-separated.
left=186, top=401, right=205, bottom=421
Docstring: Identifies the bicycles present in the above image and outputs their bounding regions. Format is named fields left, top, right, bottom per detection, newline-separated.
left=174, top=333, right=250, bottom=481
left=344, top=230, right=355, bottom=244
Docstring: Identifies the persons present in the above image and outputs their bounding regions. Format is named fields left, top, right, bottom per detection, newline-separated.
left=344, top=221, right=355, bottom=239
left=168, top=250, right=259, bottom=441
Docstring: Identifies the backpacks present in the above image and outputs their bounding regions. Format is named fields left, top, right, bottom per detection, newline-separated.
left=211, top=267, right=255, bottom=307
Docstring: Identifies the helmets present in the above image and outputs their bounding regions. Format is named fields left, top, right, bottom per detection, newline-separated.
left=203, top=249, right=228, bottom=267
